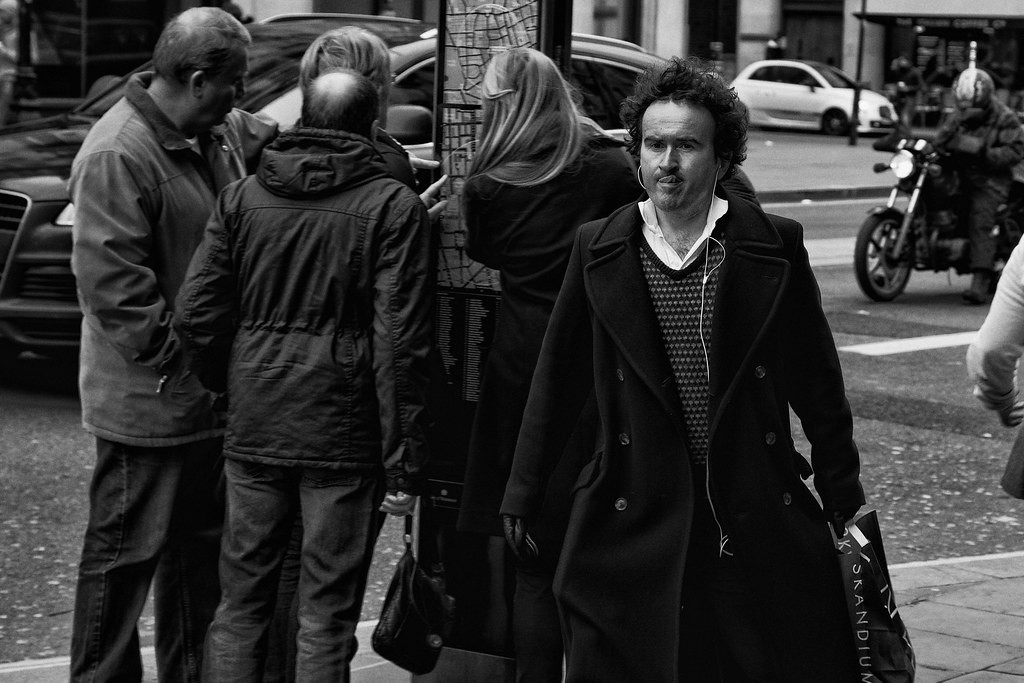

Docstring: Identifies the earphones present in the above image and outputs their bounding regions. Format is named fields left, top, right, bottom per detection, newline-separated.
left=719, top=161, right=721, bottom=168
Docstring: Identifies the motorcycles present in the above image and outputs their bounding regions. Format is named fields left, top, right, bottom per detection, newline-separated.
left=853, top=106, right=1024, bottom=303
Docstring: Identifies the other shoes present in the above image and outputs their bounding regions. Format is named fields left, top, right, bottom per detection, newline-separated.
left=969, top=272, right=992, bottom=305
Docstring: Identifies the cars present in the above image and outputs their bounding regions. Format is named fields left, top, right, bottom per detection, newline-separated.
left=0, top=12, right=670, bottom=359
left=731, top=59, right=901, bottom=136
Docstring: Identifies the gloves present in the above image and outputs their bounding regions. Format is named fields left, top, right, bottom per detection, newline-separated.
left=826, top=506, right=861, bottom=541
left=502, top=514, right=526, bottom=557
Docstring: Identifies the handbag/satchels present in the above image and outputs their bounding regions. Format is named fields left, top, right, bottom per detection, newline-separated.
left=371, top=551, right=458, bottom=677
left=830, top=508, right=916, bottom=683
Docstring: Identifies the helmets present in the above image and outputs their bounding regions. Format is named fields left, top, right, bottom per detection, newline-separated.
left=950, top=67, right=995, bottom=117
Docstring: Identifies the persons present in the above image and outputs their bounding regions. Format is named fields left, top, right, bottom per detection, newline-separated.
left=891, top=53, right=1024, bottom=499
left=451, top=46, right=640, bottom=682
left=499, top=56, right=865, bottom=683
left=66, top=6, right=447, bottom=683
